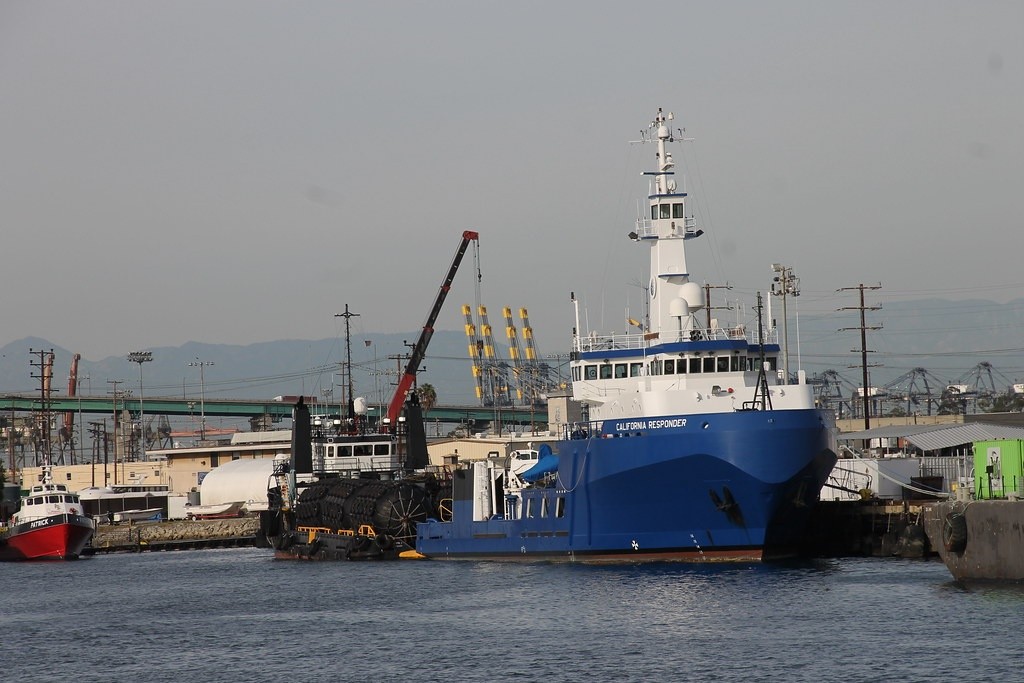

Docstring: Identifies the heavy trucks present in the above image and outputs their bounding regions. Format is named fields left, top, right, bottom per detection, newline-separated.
left=274, top=396, right=318, bottom=403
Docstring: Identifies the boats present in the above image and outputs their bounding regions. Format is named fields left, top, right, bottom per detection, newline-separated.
left=78, top=474, right=188, bottom=520
left=258, top=463, right=448, bottom=561
left=92, top=513, right=108, bottom=524
left=398, top=106, right=842, bottom=564
left=0, top=455, right=96, bottom=560
left=183, top=501, right=246, bottom=516
left=107, top=508, right=163, bottom=522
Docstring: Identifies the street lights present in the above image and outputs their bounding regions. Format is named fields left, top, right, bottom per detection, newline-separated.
left=68, top=376, right=89, bottom=465
left=127, top=352, right=153, bottom=462
left=771, top=263, right=788, bottom=386
left=187, top=363, right=215, bottom=440
